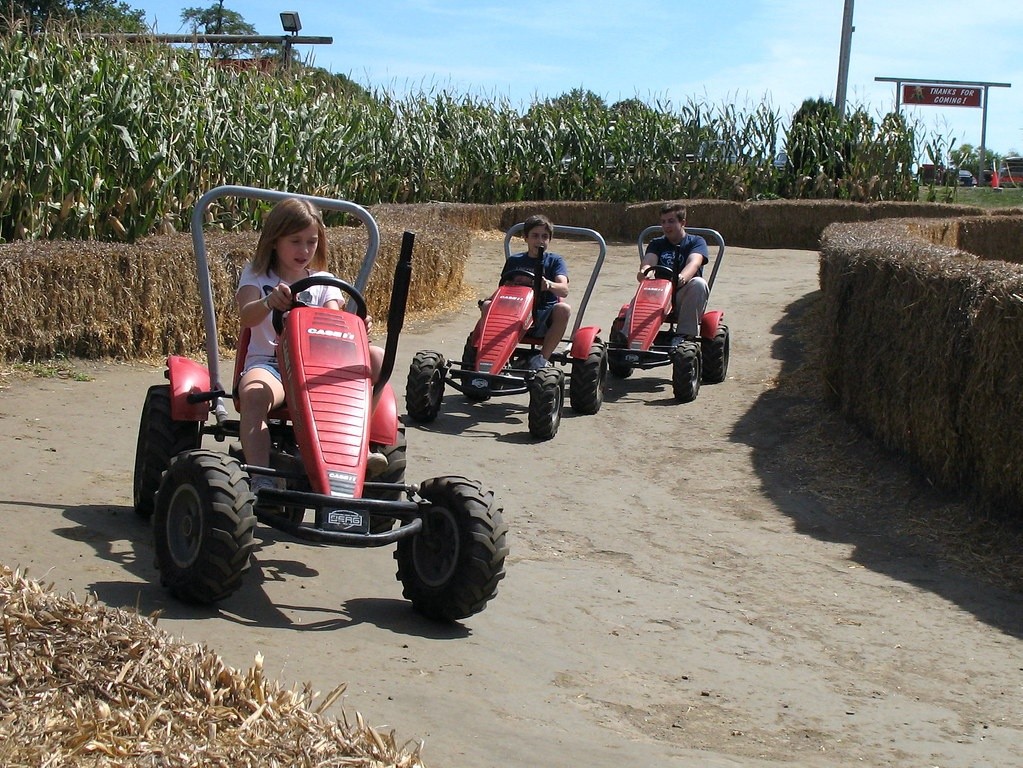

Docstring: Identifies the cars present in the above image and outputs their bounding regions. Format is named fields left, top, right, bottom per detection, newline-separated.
left=942, top=168, right=977, bottom=187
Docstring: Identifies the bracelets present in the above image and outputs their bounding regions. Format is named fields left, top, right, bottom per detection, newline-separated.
left=263, top=296, right=273, bottom=310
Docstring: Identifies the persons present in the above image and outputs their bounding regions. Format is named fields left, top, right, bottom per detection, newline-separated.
left=481, top=214, right=571, bottom=380
left=636, top=201, right=710, bottom=354
left=236, top=206, right=389, bottom=495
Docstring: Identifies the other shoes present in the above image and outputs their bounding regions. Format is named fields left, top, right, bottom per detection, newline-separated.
left=669, top=336, right=683, bottom=353
left=531, top=354, right=551, bottom=370
left=250, top=477, right=281, bottom=507
left=366, top=450, right=388, bottom=479
